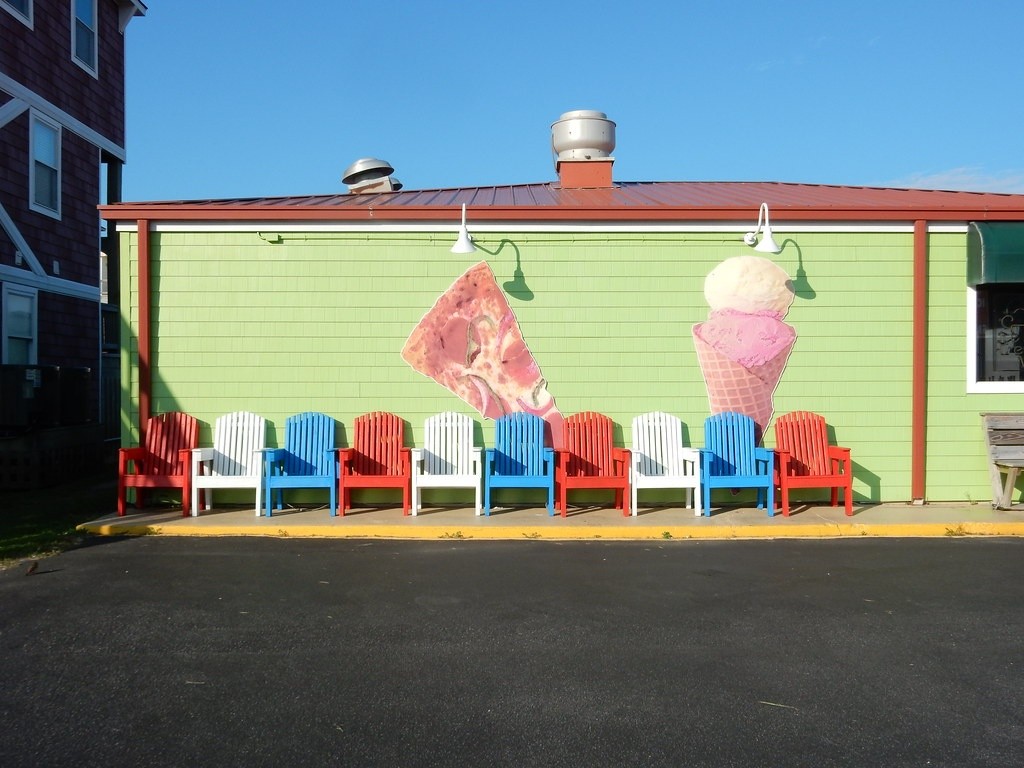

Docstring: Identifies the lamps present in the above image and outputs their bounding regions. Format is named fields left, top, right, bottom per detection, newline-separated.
left=450, top=203, right=476, bottom=254
left=746, top=202, right=778, bottom=254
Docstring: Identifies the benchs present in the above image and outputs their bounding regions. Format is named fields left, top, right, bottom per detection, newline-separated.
left=979, top=412, right=1024, bottom=512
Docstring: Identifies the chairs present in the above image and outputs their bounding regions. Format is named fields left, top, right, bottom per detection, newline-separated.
left=411, top=411, right=482, bottom=516
left=191, top=410, right=265, bottom=517
left=628, top=411, right=702, bottom=517
left=265, top=412, right=339, bottom=517
left=697, top=410, right=774, bottom=517
left=117, top=411, right=204, bottom=517
left=484, top=412, right=555, bottom=517
left=339, top=412, right=411, bottom=516
left=554, top=411, right=630, bottom=518
left=774, top=410, right=853, bottom=516
